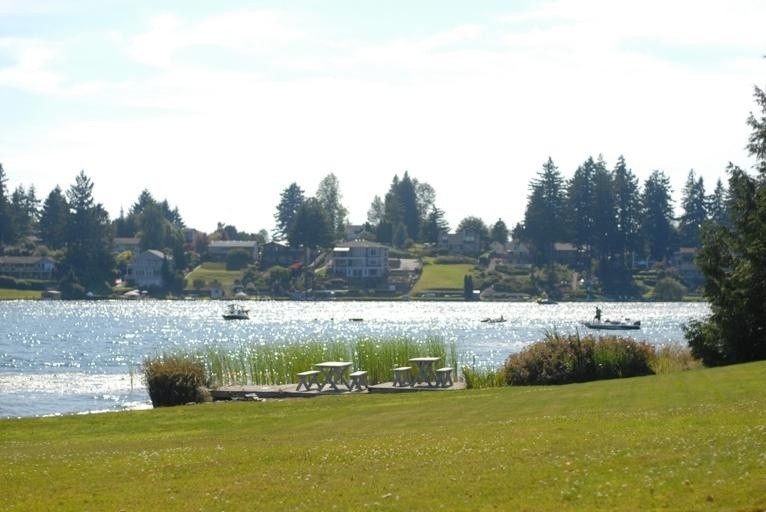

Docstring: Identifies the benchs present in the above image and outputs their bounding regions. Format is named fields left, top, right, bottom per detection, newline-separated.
left=296, top=369, right=368, bottom=392
left=391, top=366, right=454, bottom=387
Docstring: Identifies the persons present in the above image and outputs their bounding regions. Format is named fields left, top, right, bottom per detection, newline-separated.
left=593, top=307, right=602, bottom=323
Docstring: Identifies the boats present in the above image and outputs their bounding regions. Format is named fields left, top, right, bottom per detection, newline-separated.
left=222, top=303, right=250, bottom=320
left=584, top=322, right=641, bottom=329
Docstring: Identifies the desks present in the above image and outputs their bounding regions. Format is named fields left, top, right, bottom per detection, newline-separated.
left=314, top=360, right=353, bottom=392
left=408, top=356, right=440, bottom=387
